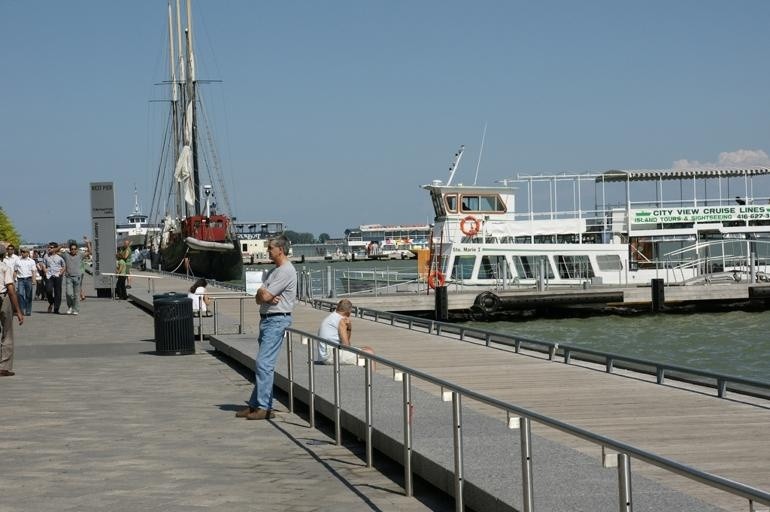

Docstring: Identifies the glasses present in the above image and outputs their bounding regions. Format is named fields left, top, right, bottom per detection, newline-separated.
left=21, top=246, right=77, bottom=253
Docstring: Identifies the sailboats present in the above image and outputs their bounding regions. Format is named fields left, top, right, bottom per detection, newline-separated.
left=142, top=0, right=244, bottom=283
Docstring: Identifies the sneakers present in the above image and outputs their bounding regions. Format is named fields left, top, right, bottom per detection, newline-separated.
left=65, top=307, right=80, bottom=315
left=235, top=405, right=276, bottom=420
left=47, top=303, right=62, bottom=316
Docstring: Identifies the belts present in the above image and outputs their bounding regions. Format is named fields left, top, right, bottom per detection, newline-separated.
left=260, top=312, right=292, bottom=318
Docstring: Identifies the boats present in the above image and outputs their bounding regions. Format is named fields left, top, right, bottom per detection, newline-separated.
left=231, top=220, right=294, bottom=259
left=117, top=183, right=162, bottom=255
left=343, top=223, right=435, bottom=256
left=339, top=143, right=770, bottom=294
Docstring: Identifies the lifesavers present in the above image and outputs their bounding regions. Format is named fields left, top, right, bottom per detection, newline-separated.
left=429, top=272, right=444, bottom=288
left=461, top=216, right=479, bottom=235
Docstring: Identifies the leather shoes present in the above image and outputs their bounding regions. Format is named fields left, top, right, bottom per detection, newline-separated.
left=0, top=370, right=16, bottom=378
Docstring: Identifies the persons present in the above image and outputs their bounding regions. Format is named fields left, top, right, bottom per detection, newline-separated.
left=188, top=279, right=212, bottom=317
left=318, top=300, right=376, bottom=373
left=0, top=240, right=155, bottom=376
left=235, top=236, right=298, bottom=420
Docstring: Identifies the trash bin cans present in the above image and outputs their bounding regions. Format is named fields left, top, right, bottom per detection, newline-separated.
left=152, top=292, right=195, bottom=355
